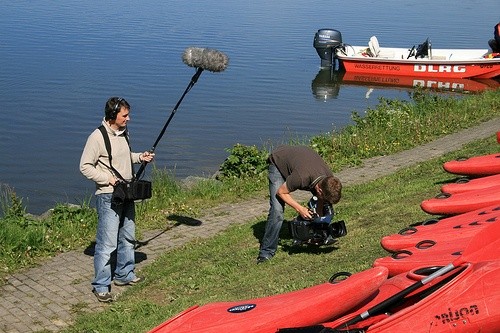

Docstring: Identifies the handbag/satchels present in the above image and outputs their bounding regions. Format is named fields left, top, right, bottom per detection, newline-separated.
left=111, top=180, right=152, bottom=206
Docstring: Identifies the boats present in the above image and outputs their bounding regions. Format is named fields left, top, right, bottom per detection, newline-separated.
left=141, top=153, right=500, bottom=333
left=311, top=70, right=499, bottom=101
left=312, top=28, right=500, bottom=77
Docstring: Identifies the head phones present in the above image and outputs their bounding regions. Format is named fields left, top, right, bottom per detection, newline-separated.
left=109, top=98, right=124, bottom=120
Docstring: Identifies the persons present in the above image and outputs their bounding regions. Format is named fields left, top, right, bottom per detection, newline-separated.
left=256, top=146, right=342, bottom=262
left=488, top=22, right=500, bottom=54
left=79, top=98, right=156, bottom=302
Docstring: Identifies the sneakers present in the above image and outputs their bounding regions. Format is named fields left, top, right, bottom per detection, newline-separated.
left=113, top=276, right=144, bottom=286
left=93, top=288, right=112, bottom=301
left=257, top=257, right=269, bottom=265
